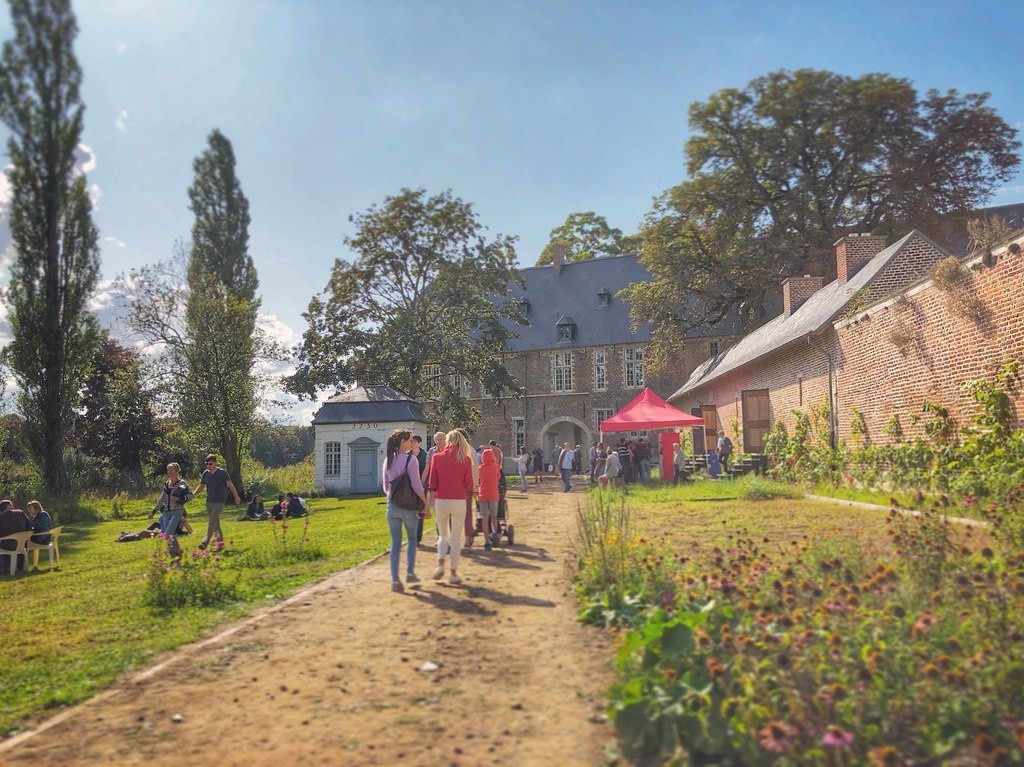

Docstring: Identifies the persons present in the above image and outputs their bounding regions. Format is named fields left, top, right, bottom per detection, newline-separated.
left=597, top=446, right=623, bottom=493
left=476, top=439, right=503, bottom=471
left=23, top=501, right=51, bottom=550
left=574, top=444, right=583, bottom=474
left=714, top=430, right=732, bottom=475
left=551, top=444, right=563, bottom=478
left=146, top=506, right=193, bottom=535
left=672, top=442, right=692, bottom=488
left=452, top=428, right=479, bottom=547
left=382, top=429, right=432, bottom=591
left=429, top=431, right=474, bottom=584
left=511, top=446, right=529, bottom=493
left=246, top=495, right=264, bottom=519
left=476, top=449, right=502, bottom=547
left=0, top=500, right=32, bottom=572
left=148, top=462, right=194, bottom=564
left=420, top=432, right=451, bottom=547
left=533, top=444, right=544, bottom=482
left=272, top=491, right=310, bottom=518
left=402, top=434, right=429, bottom=549
left=589, top=441, right=607, bottom=484
left=615, top=436, right=652, bottom=489
left=191, top=453, right=242, bottom=551
left=557, top=441, right=575, bottom=492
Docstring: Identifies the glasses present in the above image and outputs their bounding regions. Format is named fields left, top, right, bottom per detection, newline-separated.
left=205, top=462, right=213, bottom=465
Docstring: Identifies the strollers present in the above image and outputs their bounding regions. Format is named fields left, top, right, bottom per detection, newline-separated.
left=473, top=493, right=515, bottom=544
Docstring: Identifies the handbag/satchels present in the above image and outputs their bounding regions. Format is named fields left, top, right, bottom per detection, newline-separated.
left=173, top=484, right=190, bottom=504
left=388, top=473, right=425, bottom=511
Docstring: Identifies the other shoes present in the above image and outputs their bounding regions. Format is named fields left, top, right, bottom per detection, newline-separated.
left=450, top=575, right=462, bottom=583
left=484, top=542, right=492, bottom=550
left=432, top=566, right=445, bottom=580
left=492, top=533, right=498, bottom=541
left=392, top=580, right=404, bottom=591
left=405, top=574, right=421, bottom=583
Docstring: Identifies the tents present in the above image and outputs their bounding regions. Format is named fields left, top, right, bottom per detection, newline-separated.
left=601, top=385, right=709, bottom=476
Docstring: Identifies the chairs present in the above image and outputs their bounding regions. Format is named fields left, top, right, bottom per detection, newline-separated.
left=27, top=525, right=65, bottom=572
left=0, top=530, right=35, bottom=577
